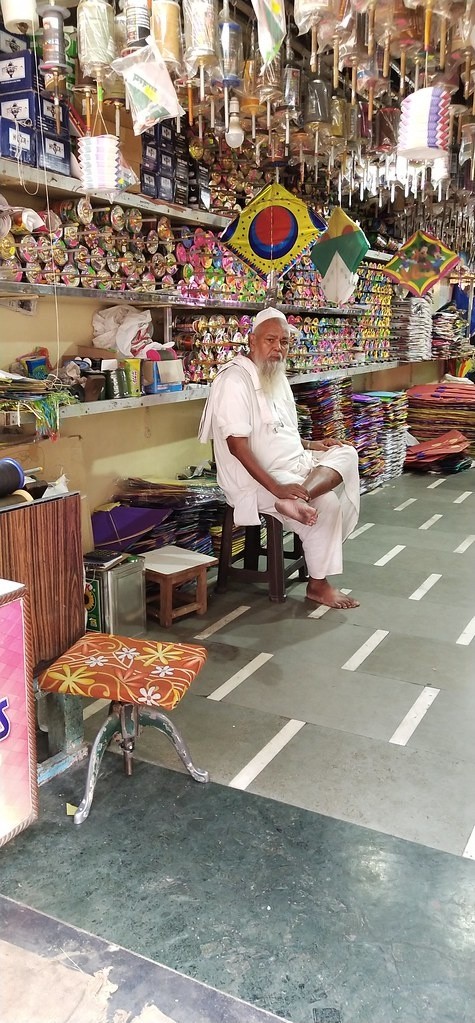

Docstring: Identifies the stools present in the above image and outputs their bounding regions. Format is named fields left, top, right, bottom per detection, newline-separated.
left=37, top=633, right=206, bottom=823
left=137, top=546, right=218, bottom=626
left=217, top=503, right=308, bottom=605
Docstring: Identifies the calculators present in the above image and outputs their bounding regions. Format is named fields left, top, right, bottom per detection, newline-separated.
left=84, top=547, right=121, bottom=562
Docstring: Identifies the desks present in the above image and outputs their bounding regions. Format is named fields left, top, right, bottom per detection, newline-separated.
left=0, top=491, right=85, bottom=677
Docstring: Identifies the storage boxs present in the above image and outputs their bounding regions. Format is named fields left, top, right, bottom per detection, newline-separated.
left=140, top=360, right=186, bottom=394
left=0, top=26, right=210, bottom=212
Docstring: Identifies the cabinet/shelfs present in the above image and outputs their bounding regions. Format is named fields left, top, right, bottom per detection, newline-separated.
left=0, top=159, right=475, bottom=427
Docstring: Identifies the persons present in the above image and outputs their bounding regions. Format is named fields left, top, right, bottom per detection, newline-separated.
left=197, top=308, right=360, bottom=609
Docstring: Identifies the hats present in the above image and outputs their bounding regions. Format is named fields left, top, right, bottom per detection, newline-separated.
left=252, top=307, right=287, bottom=333
